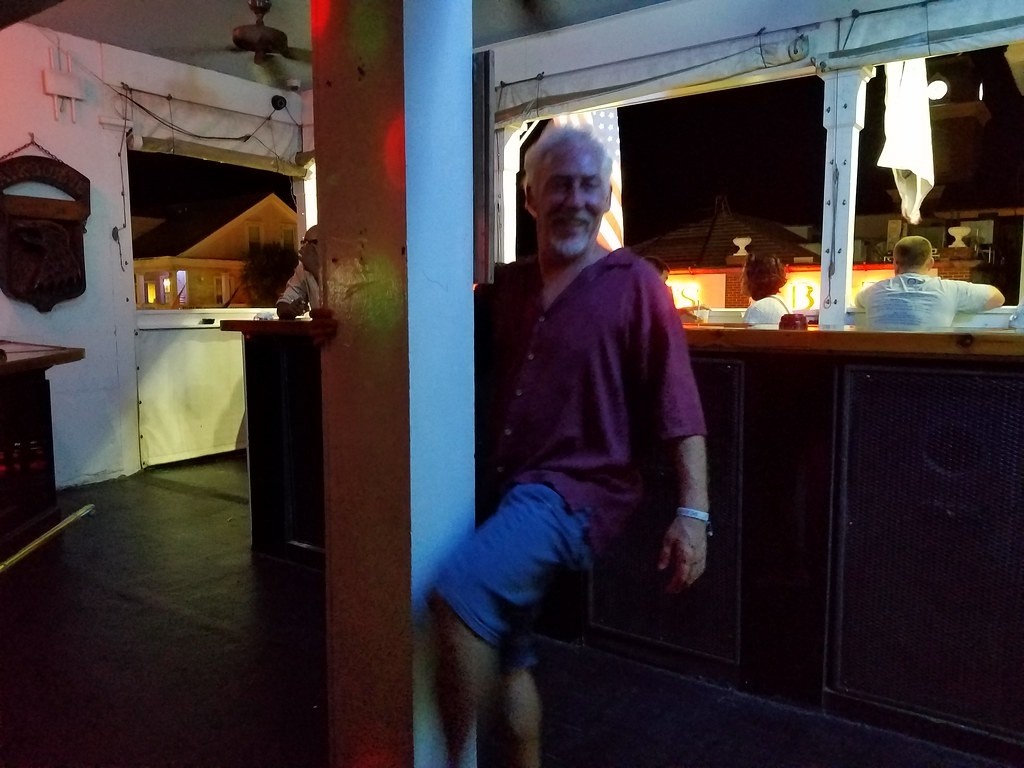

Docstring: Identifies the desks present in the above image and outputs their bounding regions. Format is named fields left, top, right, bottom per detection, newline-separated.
left=0, top=339, right=85, bottom=578
left=220, top=318, right=324, bottom=558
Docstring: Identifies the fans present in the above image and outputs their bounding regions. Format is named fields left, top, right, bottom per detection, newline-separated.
left=131, top=0, right=311, bottom=89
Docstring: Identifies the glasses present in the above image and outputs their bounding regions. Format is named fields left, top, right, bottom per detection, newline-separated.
left=301, top=240, right=318, bottom=245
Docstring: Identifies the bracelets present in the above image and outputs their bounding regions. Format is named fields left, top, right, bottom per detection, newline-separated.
left=676, top=506, right=709, bottom=521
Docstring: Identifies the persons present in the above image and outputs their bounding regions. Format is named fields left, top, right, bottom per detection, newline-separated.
left=438, top=127, right=710, bottom=767
left=275, top=224, right=321, bottom=319
left=854, top=236, right=1005, bottom=328
left=739, top=255, right=793, bottom=323
left=640, top=256, right=712, bottom=324
